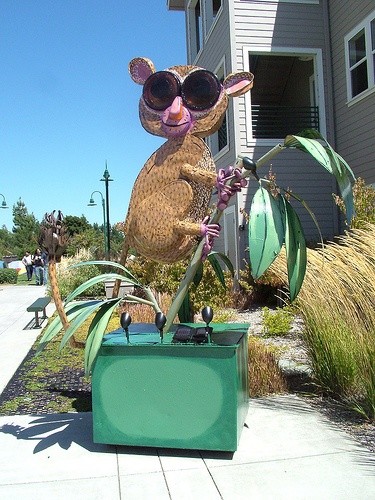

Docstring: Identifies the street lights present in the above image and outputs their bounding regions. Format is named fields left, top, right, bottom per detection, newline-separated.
left=0, top=192, right=8, bottom=209
left=88, top=158, right=114, bottom=273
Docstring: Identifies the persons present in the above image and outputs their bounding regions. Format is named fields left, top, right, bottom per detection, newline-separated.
left=22, top=251, right=34, bottom=281
left=34, top=248, right=47, bottom=287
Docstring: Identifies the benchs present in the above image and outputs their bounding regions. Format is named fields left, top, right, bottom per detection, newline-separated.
left=26, top=297, right=52, bottom=329
